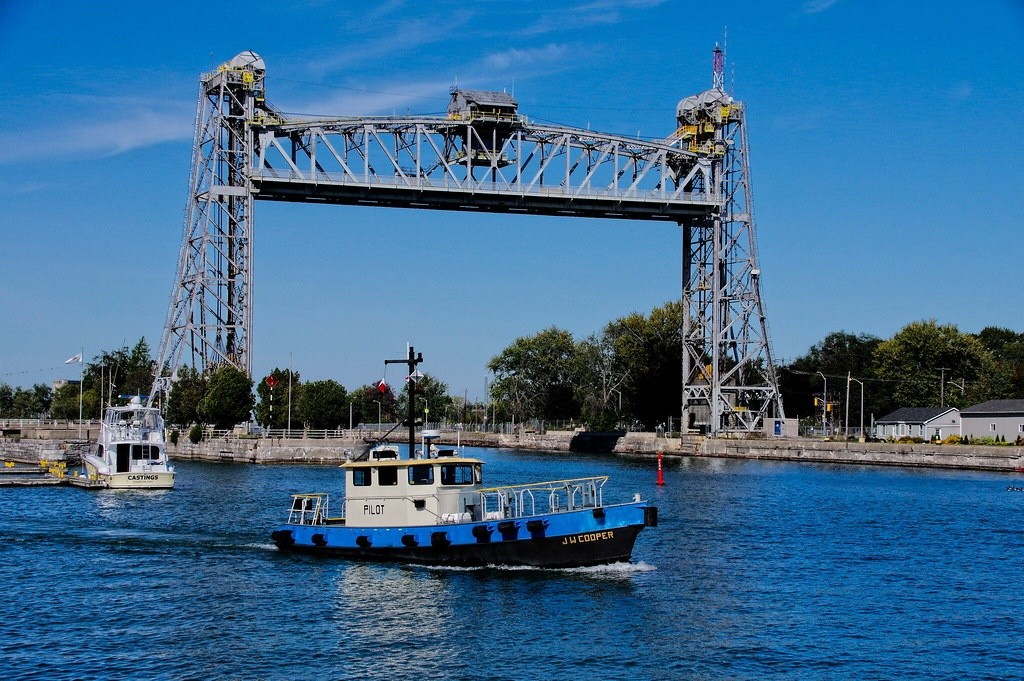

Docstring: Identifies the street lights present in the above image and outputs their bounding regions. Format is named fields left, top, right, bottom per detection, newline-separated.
left=349, top=397, right=358, bottom=430
left=817, top=371, right=826, bottom=436
left=610, top=387, right=621, bottom=411
left=849, top=378, right=864, bottom=438
left=373, top=400, right=381, bottom=432
left=419, top=397, right=427, bottom=430
left=947, top=378, right=965, bottom=397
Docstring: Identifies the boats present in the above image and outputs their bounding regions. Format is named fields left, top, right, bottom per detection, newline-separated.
left=83, top=395, right=177, bottom=490
left=270, top=346, right=657, bottom=568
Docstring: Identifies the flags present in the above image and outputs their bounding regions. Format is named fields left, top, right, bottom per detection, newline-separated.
left=66, top=353, right=82, bottom=364
left=376, top=379, right=386, bottom=394
